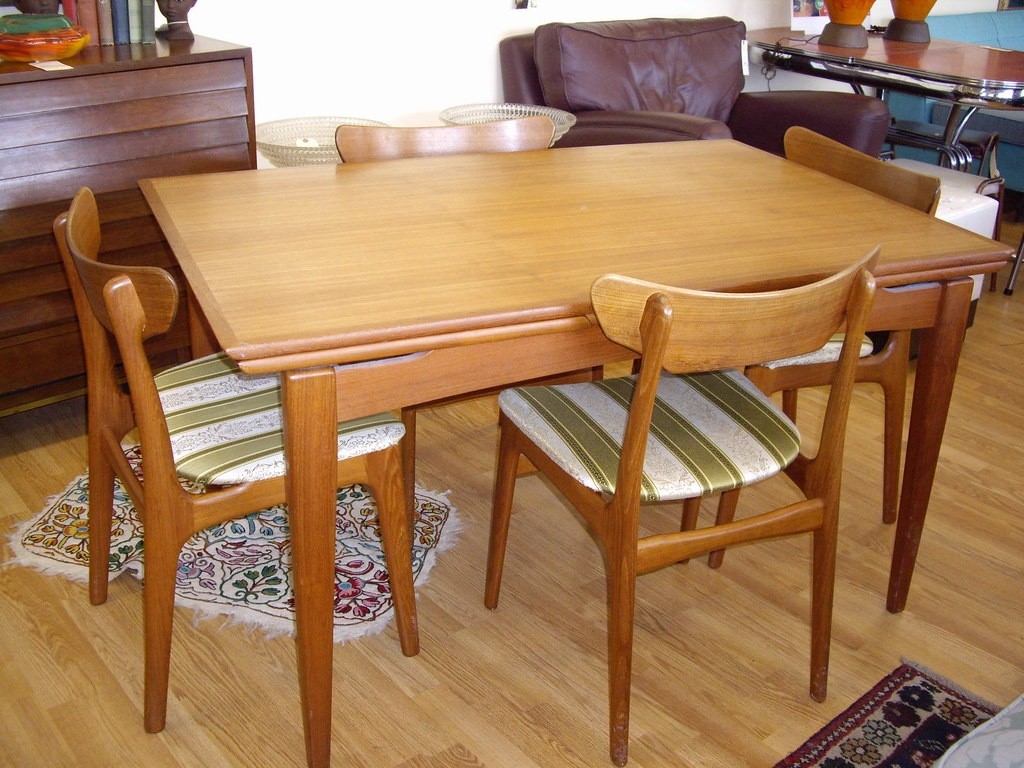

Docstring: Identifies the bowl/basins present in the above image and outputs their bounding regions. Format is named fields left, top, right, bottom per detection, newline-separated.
left=256, top=117, right=390, bottom=168
left=439, top=103, right=576, bottom=148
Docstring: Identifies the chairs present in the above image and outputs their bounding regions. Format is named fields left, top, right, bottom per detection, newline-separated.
left=52, top=189, right=422, bottom=768
left=334, top=114, right=607, bottom=547
left=705, top=126, right=940, bottom=570
left=488, top=246, right=884, bottom=767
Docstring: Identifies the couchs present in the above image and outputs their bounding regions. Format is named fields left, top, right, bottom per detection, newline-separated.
left=500, top=15, right=886, bottom=158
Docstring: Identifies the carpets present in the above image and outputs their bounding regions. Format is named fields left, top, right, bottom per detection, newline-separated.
left=13, top=442, right=465, bottom=644
left=776, top=656, right=1000, bottom=767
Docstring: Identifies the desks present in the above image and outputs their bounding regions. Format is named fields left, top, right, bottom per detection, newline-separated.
left=0, top=42, right=257, bottom=394
left=139, top=137, right=1016, bottom=767
left=740, top=24, right=1024, bottom=143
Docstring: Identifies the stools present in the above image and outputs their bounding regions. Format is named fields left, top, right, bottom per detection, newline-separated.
left=861, top=160, right=1005, bottom=361
